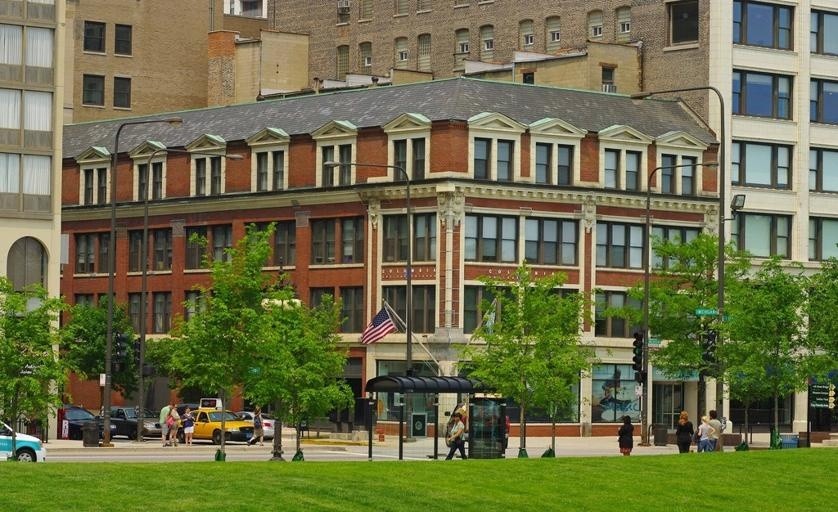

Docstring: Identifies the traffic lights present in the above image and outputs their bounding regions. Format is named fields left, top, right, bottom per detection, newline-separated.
left=631, top=328, right=643, bottom=371
left=700, top=327, right=718, bottom=364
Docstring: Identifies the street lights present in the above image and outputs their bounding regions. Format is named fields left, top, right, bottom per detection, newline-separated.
left=324, top=158, right=416, bottom=446
left=628, top=85, right=731, bottom=451
left=639, top=160, right=722, bottom=445
left=136, top=147, right=243, bottom=442
left=100, top=115, right=183, bottom=448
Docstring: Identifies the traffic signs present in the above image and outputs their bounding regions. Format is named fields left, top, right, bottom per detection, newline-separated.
left=695, top=308, right=718, bottom=317
left=647, top=338, right=661, bottom=344
left=809, top=383, right=836, bottom=411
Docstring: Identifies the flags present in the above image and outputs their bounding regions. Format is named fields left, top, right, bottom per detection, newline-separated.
left=482, top=303, right=496, bottom=346
left=360, top=307, right=399, bottom=346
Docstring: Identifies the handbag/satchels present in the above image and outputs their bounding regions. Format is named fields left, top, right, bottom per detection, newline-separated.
left=455, top=439, right=463, bottom=447
left=255, top=419, right=261, bottom=428
left=167, top=416, right=174, bottom=426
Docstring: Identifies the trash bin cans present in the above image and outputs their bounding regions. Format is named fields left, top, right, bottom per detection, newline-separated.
left=653, top=423, right=667, bottom=446
left=799, top=432, right=811, bottom=447
left=781, top=435, right=799, bottom=449
left=82, top=422, right=100, bottom=447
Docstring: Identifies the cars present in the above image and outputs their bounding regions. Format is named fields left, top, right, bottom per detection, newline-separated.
left=445, top=399, right=510, bottom=449
left=0, top=420, right=46, bottom=463
left=58, top=396, right=278, bottom=447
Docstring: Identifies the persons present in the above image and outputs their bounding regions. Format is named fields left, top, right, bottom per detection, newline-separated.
left=617, top=415, right=634, bottom=456
left=675, top=411, right=694, bottom=453
left=165, top=408, right=181, bottom=447
left=697, top=416, right=716, bottom=453
left=158, top=401, right=175, bottom=448
left=445, top=412, right=467, bottom=461
left=247, top=407, right=264, bottom=446
left=181, top=405, right=195, bottom=446
left=708, top=410, right=721, bottom=451
